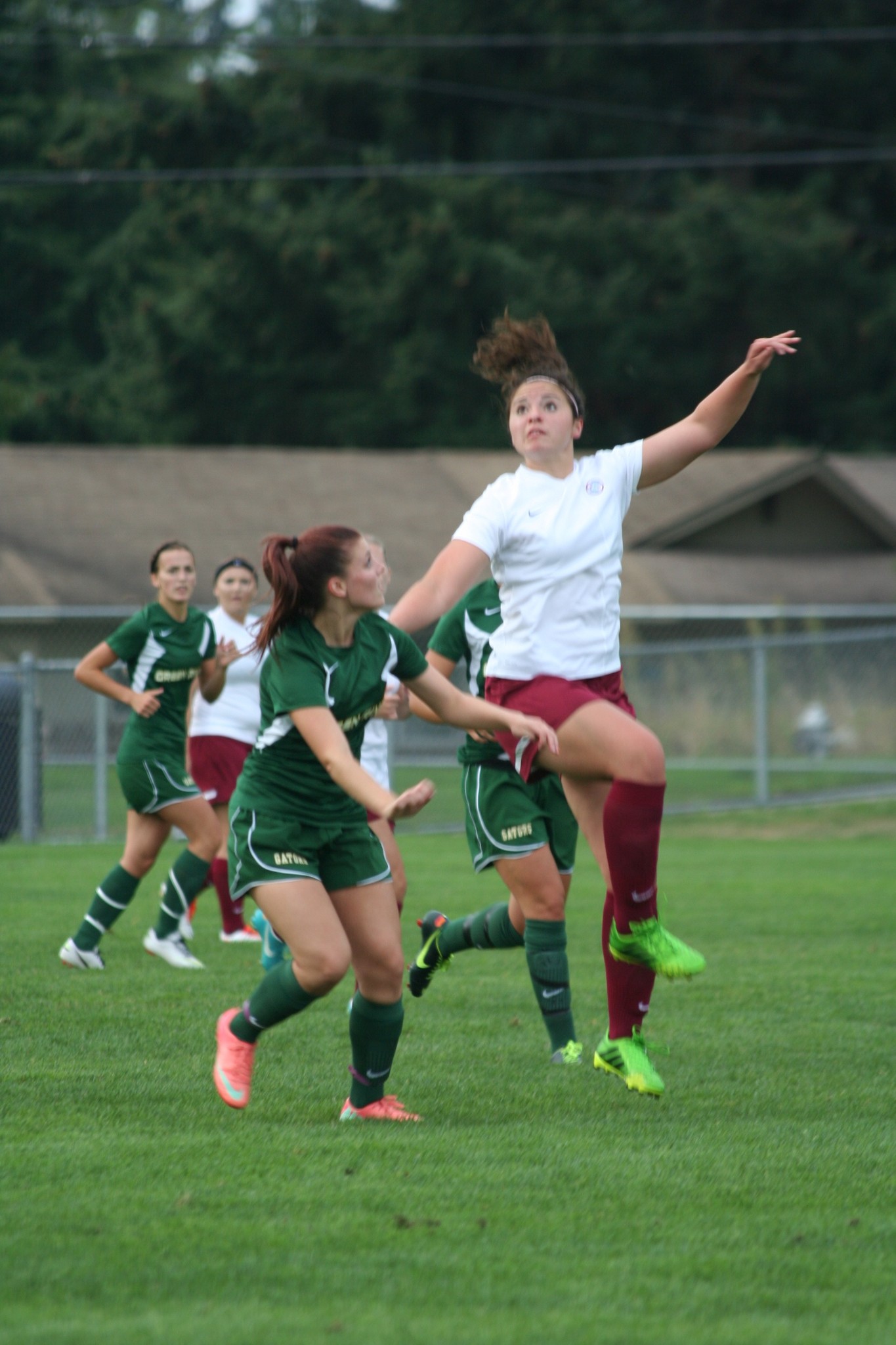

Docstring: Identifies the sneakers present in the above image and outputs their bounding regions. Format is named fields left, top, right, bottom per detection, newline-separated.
left=219, top=925, right=263, bottom=942
left=160, top=883, right=196, bottom=940
left=251, top=909, right=284, bottom=972
left=213, top=1008, right=256, bottom=1109
left=339, top=1096, right=424, bottom=1123
left=551, top=1040, right=584, bottom=1065
left=406, top=911, right=454, bottom=997
left=594, top=1024, right=670, bottom=1101
left=143, top=928, right=205, bottom=969
left=609, top=916, right=706, bottom=981
left=59, top=937, right=104, bottom=970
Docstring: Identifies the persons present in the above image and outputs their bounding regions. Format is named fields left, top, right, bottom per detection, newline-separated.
left=175, top=557, right=260, bottom=946
left=251, top=571, right=593, bottom=1071
left=59, top=541, right=240, bottom=970
left=212, top=524, right=562, bottom=1121
left=386, top=305, right=800, bottom=1100
